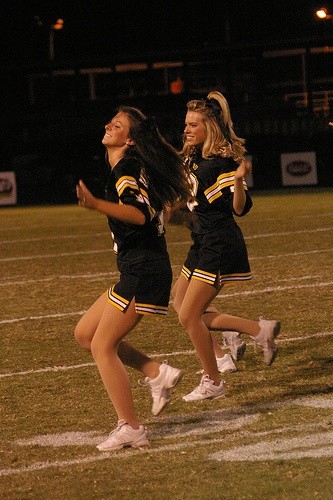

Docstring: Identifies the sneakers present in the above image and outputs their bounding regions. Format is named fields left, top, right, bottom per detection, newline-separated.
left=183, top=375, right=225, bottom=402
left=219, top=331, right=246, bottom=361
left=137, top=360, right=183, bottom=416
left=195, top=354, right=237, bottom=373
left=96, top=419, right=149, bottom=452
left=250, top=316, right=280, bottom=365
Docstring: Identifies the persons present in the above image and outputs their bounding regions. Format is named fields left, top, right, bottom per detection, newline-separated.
left=169, top=92, right=282, bottom=401
left=74, top=105, right=183, bottom=452
